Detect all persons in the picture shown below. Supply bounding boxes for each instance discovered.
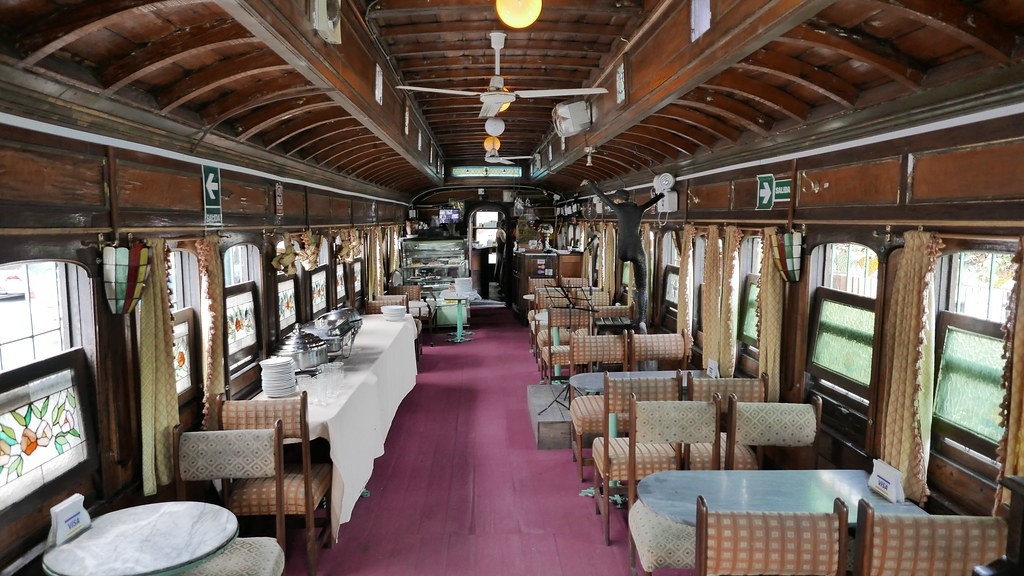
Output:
[581,180,666,334]
[495,219,517,300]
[536,225,543,249]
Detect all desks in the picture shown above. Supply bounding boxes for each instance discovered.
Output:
[40,501,241,576]
[43,289,933,544]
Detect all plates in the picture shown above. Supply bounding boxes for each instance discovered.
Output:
[454,278,473,294]
[259,357,296,397]
[380,305,407,321]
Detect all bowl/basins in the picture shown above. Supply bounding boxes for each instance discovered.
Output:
[405,234,419,238]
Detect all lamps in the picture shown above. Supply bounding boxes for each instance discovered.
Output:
[496,0,542,29]
[587,152,593,166]
[484,137,500,152]
[499,87,511,112]
[653,173,678,212]
[485,117,506,137]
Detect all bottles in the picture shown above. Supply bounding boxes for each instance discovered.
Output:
[576,239,580,247]
[570,238,575,247]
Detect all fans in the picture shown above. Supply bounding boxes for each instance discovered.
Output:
[440,136,534,165]
[395,33,609,117]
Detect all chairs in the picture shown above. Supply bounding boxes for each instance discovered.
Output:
[568,330,629,483]
[387,282,422,356]
[575,290,614,337]
[695,495,849,576]
[542,306,592,384]
[592,369,683,546]
[364,297,405,314]
[531,285,569,362]
[529,275,558,348]
[628,392,721,576]
[592,302,635,372]
[630,330,690,371]
[535,290,578,378]
[669,372,768,470]
[372,292,409,314]
[560,274,590,288]
[851,499,1010,576]
[724,393,822,469]
[173,391,333,576]
[572,286,605,307]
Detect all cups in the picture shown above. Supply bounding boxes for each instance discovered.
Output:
[529,240,537,249]
[443,230,449,236]
[296,362,344,408]
